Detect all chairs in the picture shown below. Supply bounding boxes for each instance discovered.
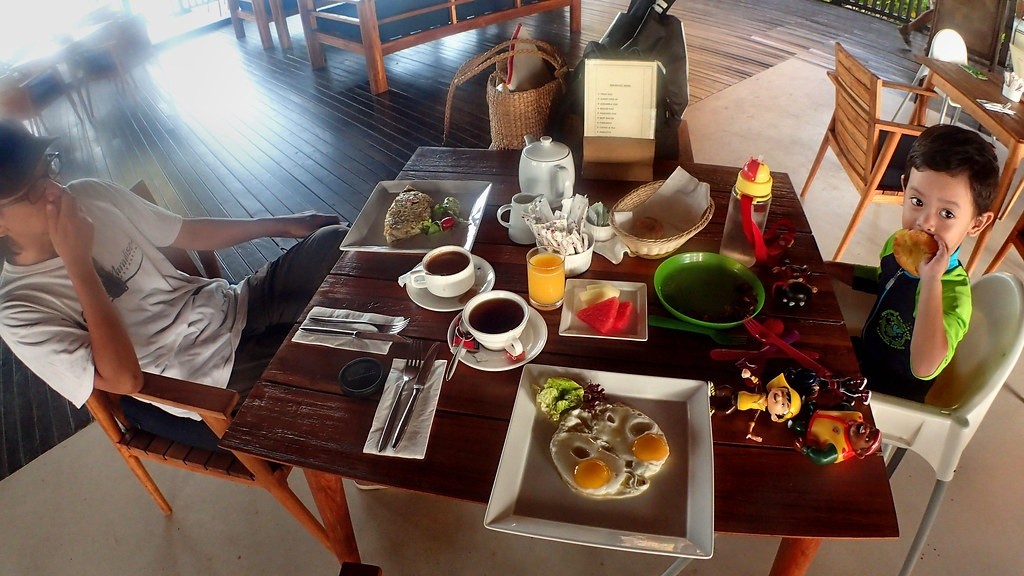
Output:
[78,179,360,566]
[1,38,136,137]
[798,29,1024,576]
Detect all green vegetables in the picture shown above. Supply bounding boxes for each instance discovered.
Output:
[533,377,607,420]
[421,204,458,234]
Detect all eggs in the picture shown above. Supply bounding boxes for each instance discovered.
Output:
[551,402,670,500]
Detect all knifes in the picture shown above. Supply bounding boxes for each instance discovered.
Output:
[391,342,440,451]
[710,349,819,360]
[300,327,414,343]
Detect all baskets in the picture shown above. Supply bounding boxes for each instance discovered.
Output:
[608,180,715,259]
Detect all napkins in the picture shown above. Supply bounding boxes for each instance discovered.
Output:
[977,99,1016,116]
[363,357,447,461]
[293,306,404,356]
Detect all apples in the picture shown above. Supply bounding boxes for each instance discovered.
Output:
[584,285,621,304]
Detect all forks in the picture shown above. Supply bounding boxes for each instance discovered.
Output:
[650,315,747,346]
[309,317,410,334]
[377,340,423,453]
[735,314,824,374]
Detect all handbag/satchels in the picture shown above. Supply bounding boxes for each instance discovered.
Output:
[566,0,689,132]
[443,38,573,150]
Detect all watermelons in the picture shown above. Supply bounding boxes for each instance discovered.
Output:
[578,297,632,334]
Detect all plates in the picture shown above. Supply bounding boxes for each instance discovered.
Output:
[484,364,715,560]
[406,254,495,312]
[447,306,548,371]
[559,278,648,342]
[339,180,492,252]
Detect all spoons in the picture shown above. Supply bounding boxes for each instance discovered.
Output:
[446,324,473,381]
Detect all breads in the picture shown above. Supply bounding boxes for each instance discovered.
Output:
[892,229,938,279]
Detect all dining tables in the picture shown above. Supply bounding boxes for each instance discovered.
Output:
[218,148,902,576]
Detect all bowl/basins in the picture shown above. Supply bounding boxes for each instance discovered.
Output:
[653,252,765,329]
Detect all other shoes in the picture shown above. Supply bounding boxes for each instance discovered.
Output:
[898,24,911,48]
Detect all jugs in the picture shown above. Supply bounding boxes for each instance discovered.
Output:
[498,193,544,245]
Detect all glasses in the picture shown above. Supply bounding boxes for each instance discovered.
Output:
[0,151,63,209]
[91,257,130,298]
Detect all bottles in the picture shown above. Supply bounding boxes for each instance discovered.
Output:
[720,151,773,269]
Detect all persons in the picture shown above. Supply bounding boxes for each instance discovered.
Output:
[0,117,388,489]
[825,123,1000,404]
[708,368,801,442]
[787,396,882,465]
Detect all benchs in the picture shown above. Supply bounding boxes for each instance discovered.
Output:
[221,0,586,94]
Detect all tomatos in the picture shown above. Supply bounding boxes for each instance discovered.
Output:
[439,217,456,230]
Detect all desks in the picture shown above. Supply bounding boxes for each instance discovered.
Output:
[9,20,114,123]
[910,54,1024,283]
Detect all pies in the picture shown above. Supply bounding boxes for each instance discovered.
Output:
[385,185,431,245]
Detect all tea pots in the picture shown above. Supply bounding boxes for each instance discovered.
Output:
[518,133,575,210]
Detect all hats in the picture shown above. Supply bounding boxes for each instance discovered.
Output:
[0,117,60,200]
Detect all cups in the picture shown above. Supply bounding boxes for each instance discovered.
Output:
[526,245,566,310]
[1002,83,1022,103]
[462,290,530,357]
[782,281,812,310]
[535,228,595,277]
[338,358,384,400]
[583,212,615,241]
[410,245,475,298]
